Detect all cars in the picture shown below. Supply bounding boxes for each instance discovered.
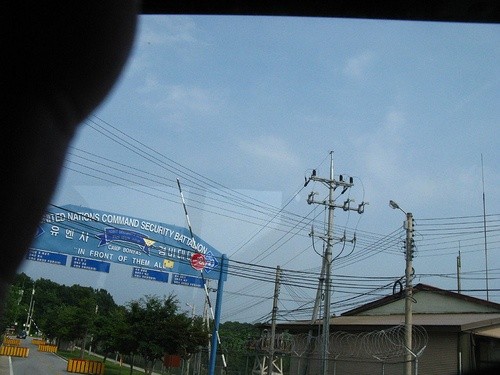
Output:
[17,331,25,338]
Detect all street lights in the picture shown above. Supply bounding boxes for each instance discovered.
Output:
[390,201,415,374]
